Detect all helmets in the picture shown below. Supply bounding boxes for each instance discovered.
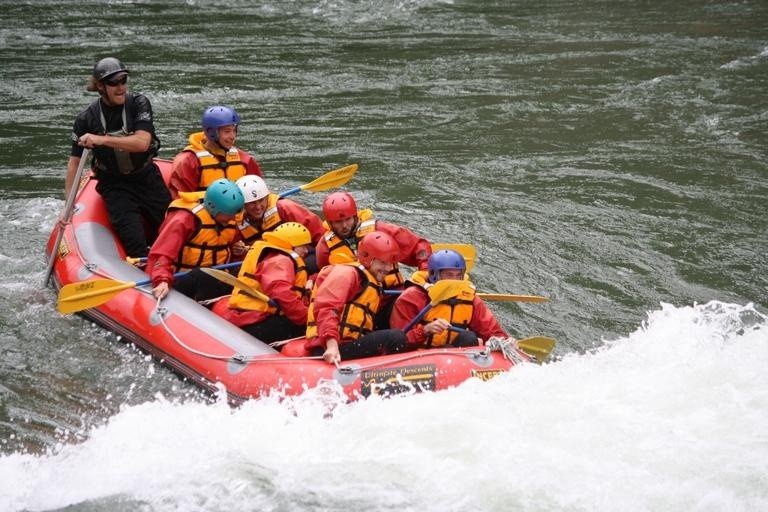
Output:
[204,179,245,217]
[428,250,465,284]
[202,106,240,143]
[272,222,312,247]
[235,175,269,203]
[358,232,401,270]
[323,192,357,230]
[93,57,130,80]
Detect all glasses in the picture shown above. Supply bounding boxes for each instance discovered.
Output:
[106,76,127,86]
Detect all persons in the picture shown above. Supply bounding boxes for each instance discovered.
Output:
[210,221,313,349]
[391,249,508,349]
[315,191,432,272]
[166,105,264,200]
[146,178,246,300]
[58,57,174,268]
[233,174,326,259]
[304,231,408,363]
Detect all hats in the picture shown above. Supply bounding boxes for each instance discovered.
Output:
[108,72,128,82]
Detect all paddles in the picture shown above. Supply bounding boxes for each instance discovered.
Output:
[278,163,356,197]
[448,325,555,364]
[58,262,245,314]
[384,289,548,304]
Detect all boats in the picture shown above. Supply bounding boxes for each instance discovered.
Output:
[44,157,530,409]
